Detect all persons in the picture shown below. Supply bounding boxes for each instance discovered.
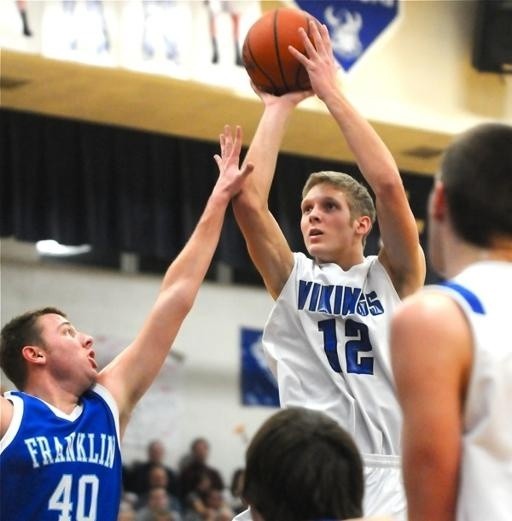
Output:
[244,405,365,521]
[116,440,244,521]
[391,122,512,521]
[2,122,254,521]
[230,20,427,521]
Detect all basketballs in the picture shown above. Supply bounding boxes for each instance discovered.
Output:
[241,9,328,93]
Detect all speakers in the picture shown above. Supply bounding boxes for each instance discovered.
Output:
[472,1,512,72]
